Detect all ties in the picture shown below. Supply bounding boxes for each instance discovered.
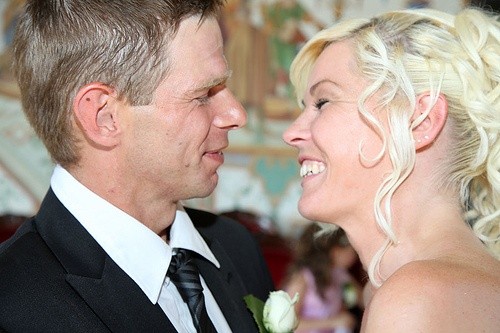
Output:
[168,249,218,333]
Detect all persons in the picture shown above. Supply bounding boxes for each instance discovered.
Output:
[281,5,500,333]
[284,226,365,333]
[0,2,275,333]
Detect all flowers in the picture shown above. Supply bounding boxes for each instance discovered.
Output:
[241,287,301,333]
